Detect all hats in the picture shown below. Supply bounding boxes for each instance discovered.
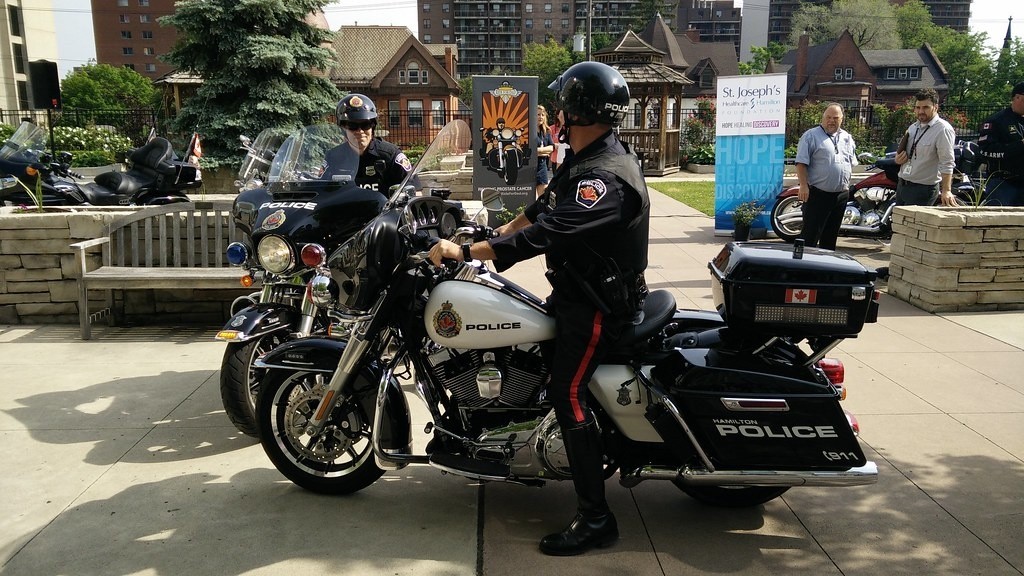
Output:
[1013,81,1024,95]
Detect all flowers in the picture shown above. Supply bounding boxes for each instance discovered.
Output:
[731,199,766,226]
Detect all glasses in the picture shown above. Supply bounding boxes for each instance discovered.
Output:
[342,120,374,131]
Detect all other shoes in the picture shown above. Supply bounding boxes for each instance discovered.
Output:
[875,267,889,279]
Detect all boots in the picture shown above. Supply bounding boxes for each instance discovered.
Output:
[539,418,619,555]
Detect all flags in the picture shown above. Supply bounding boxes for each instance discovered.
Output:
[193,132,201,157]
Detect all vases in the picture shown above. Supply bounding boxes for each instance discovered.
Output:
[733,224,751,241]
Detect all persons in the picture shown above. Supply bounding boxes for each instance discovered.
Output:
[875,89,958,281]
[316,94,423,200]
[430,62,649,556]
[550,109,570,177]
[536,105,555,197]
[794,102,859,251]
[977,81,1024,206]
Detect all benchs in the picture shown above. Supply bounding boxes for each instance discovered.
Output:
[69,201,265,341]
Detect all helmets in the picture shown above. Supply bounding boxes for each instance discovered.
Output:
[555,61,630,125]
[336,94,377,126]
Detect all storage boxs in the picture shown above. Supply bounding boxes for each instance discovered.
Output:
[157,161,204,191]
[707,241,875,336]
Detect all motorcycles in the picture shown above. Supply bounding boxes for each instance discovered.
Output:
[0,120,204,206]
[769,139,986,254]
[215,121,451,435]
[252,119,892,576]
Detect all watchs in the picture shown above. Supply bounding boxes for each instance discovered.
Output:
[461,243,473,263]
